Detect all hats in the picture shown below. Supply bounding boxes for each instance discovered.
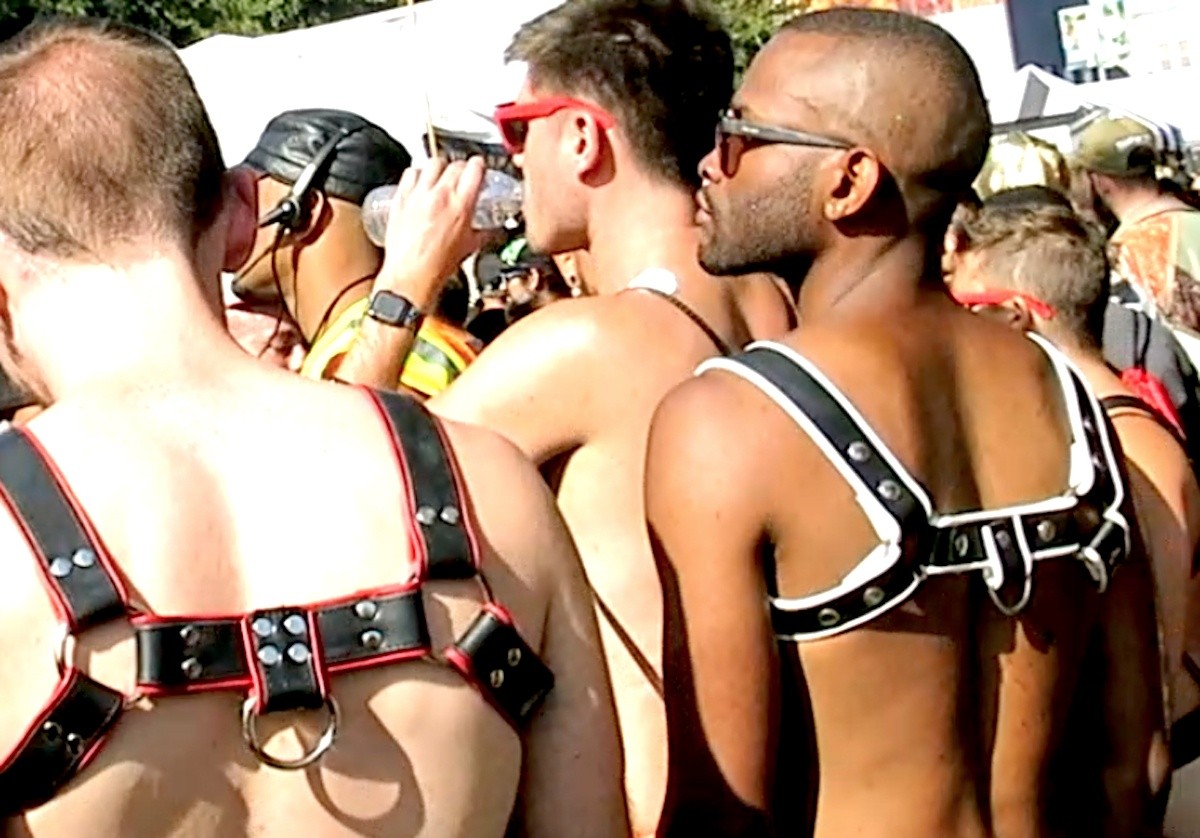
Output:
[1072,118,1157,178]
[245,105,412,206]
[500,238,549,267]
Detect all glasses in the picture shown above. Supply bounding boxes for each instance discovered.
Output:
[499,271,527,283]
[714,112,862,175]
[490,101,617,150]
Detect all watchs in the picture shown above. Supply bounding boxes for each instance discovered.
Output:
[364,289,429,337]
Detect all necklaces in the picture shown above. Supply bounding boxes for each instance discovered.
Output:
[298,272,376,375]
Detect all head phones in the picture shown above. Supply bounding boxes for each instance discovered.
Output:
[277,121,373,228]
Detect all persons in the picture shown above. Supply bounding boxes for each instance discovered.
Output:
[1,1,1200,838]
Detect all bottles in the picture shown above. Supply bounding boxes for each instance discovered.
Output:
[362,170,523,247]
